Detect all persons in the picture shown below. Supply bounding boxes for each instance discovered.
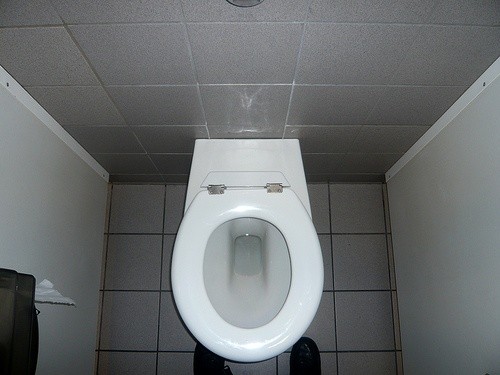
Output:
[193,337,321,375]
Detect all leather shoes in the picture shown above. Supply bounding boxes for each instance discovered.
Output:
[290,337,321,374]
[193,343,224,374]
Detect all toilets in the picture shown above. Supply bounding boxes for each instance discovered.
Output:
[170,139,325,363]
